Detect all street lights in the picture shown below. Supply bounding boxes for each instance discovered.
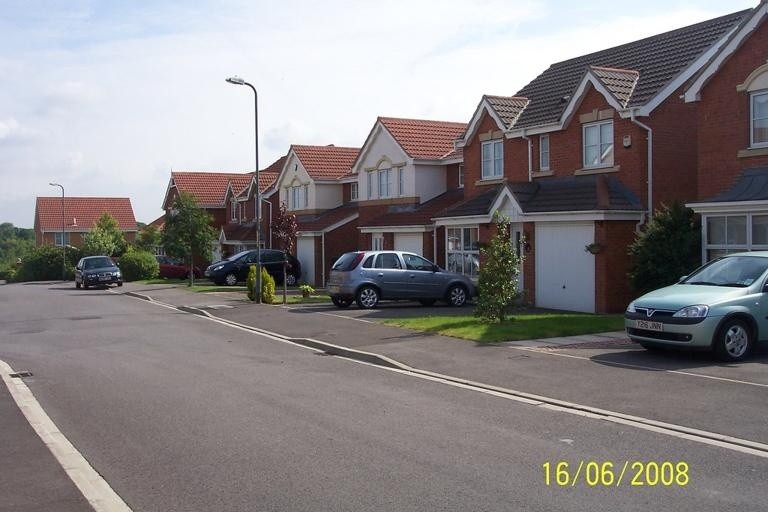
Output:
[49,183,67,281]
[226,76,262,304]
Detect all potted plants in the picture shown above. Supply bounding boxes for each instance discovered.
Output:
[584,242,603,254]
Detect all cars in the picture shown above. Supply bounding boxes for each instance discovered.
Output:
[74,255,123,288]
[204,248,301,286]
[624,251,768,363]
[154,255,202,279]
[327,250,476,308]
